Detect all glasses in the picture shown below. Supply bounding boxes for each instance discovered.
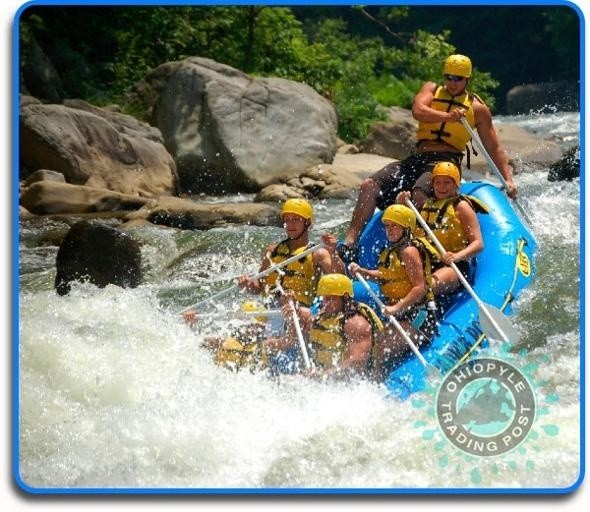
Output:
[445,74,463,82]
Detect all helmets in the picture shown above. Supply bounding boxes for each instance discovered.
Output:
[281,198,312,221]
[381,204,416,234]
[431,162,461,189]
[241,301,268,326]
[316,273,355,299]
[444,54,472,78]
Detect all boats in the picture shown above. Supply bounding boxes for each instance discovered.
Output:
[213,177,539,406]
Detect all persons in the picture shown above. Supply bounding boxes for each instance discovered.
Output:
[396,162,485,300]
[344,52,518,248]
[236,199,344,350]
[181,302,293,372]
[370,205,436,369]
[281,271,380,384]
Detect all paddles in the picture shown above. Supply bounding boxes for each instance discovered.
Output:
[404,196,520,344]
[355,272,443,387]
